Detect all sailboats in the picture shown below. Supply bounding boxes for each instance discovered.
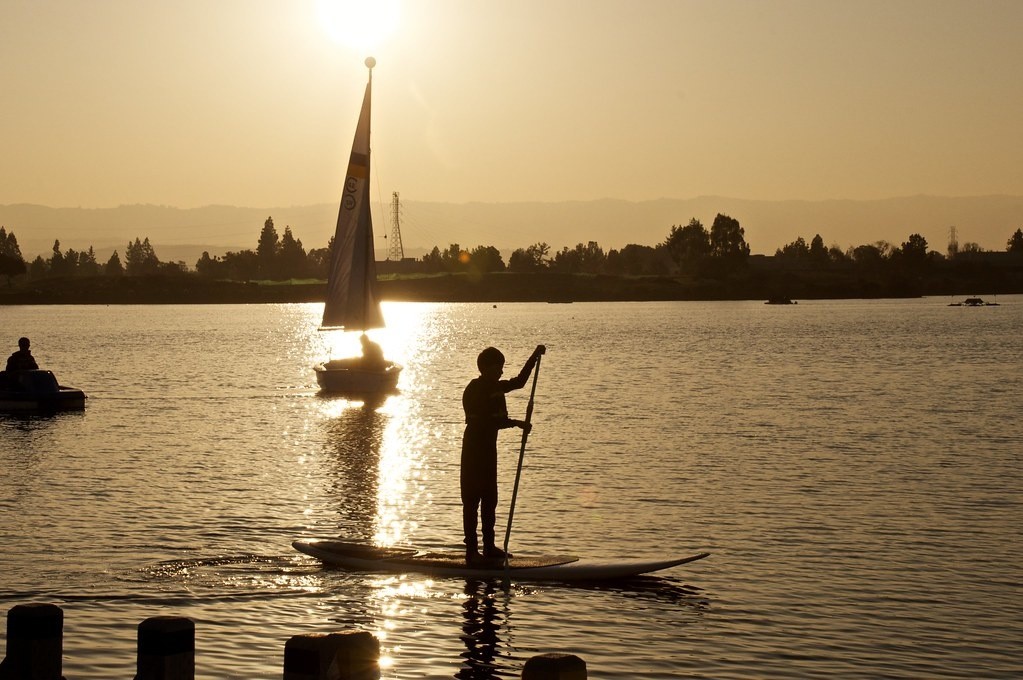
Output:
[310,56,406,390]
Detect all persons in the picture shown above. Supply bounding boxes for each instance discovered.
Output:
[356,334,386,372]
[460,345,546,565]
[5,337,39,371]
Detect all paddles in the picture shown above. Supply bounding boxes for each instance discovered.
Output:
[503,355,543,554]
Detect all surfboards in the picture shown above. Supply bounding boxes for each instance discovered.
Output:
[292,538,712,584]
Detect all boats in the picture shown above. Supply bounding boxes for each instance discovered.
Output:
[0,369,87,416]
